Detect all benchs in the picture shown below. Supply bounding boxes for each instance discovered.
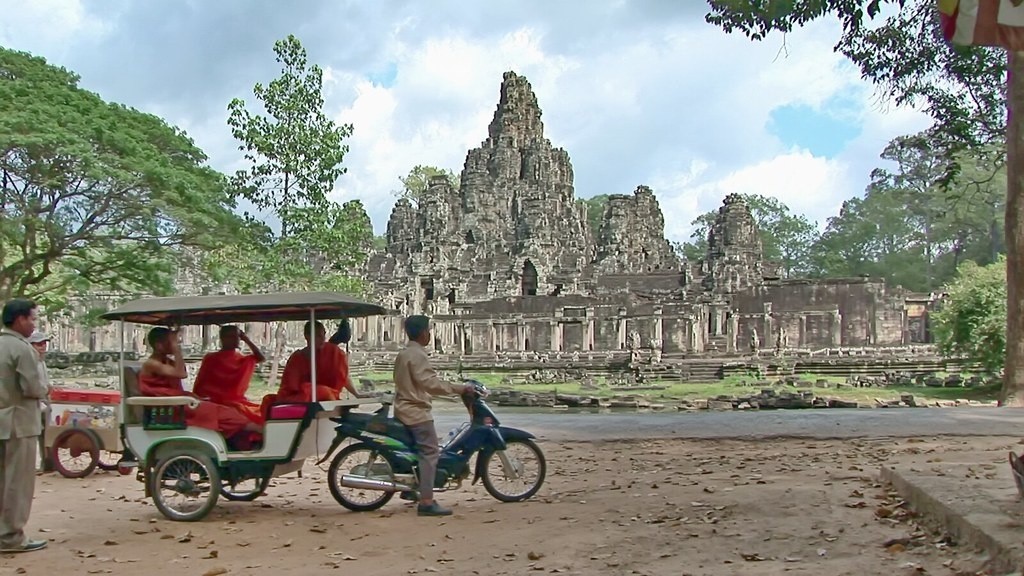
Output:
[123,365,229,453]
[263,393,394,456]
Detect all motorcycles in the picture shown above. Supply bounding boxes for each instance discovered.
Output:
[314,360,548,512]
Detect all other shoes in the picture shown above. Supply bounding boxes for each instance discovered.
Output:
[418,500,452,515]
[400,490,421,500]
[0,538,48,552]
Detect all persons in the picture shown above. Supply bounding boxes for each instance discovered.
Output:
[0,300,50,552]
[394,316,475,516]
[259,321,374,416]
[30,333,57,476]
[192,325,266,451]
[139,327,264,436]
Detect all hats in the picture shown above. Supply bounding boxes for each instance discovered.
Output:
[29,333,51,344]
